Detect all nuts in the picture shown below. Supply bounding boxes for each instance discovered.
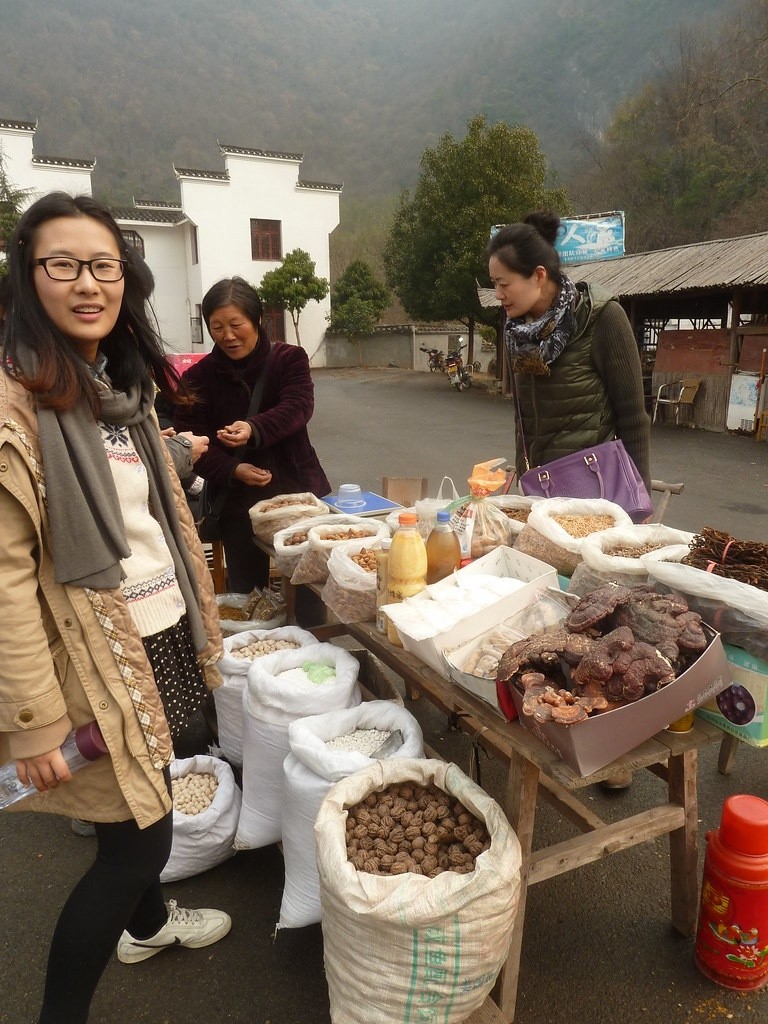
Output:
[251,500,383,626]
[171,773,221,816]
[345,781,490,879]
[227,639,303,661]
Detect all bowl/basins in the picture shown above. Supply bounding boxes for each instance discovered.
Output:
[335,483,366,509]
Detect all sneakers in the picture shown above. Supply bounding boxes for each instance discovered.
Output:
[116,898,232,964]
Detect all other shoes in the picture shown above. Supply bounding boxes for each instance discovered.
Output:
[600,771,633,789]
[71,819,95,836]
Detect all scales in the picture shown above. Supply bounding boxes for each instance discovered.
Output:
[319,491,406,524]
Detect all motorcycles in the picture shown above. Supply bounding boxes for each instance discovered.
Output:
[419,342,447,373]
[442,336,474,393]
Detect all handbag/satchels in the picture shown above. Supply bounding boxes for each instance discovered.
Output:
[413,475,461,548]
[195,478,224,542]
[518,436,654,524]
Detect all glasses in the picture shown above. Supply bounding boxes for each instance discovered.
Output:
[28,254,129,283]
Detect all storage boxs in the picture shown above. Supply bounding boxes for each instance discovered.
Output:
[392,545,768,776]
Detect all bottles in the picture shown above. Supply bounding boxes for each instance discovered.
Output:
[692,795,768,990]
[0,719,110,810]
[375,538,393,634]
[387,513,428,645]
[426,511,461,584]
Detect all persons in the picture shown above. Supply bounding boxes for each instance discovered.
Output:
[1,192,232,1023]
[486,212,650,787]
[171,276,331,594]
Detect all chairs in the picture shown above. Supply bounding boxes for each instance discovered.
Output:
[653,380,701,426]
